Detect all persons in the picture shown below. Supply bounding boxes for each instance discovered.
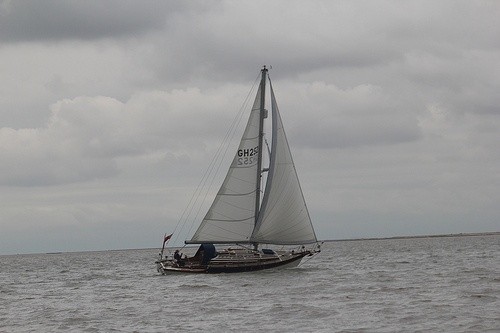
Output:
[174,250,183,261]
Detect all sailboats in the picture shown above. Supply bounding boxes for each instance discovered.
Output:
[155,65,322,276]
